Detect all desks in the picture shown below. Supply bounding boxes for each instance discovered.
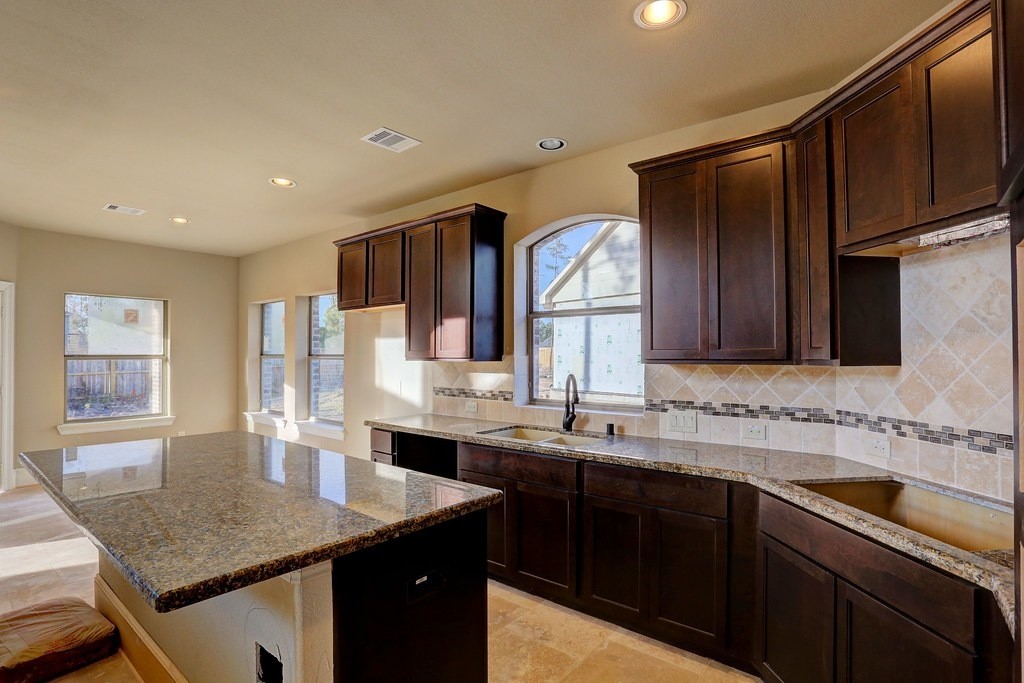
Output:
[14,430,505,683]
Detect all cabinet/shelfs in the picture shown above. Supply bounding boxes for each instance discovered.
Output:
[370,428,458,480]
[333,200,506,364]
[457,440,581,614]
[628,0,1024,365]
[751,482,994,683]
[582,459,730,666]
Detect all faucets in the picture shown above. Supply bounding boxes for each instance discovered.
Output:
[562,374,580,432]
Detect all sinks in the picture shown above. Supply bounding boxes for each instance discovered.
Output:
[541,431,609,447]
[476,424,562,442]
